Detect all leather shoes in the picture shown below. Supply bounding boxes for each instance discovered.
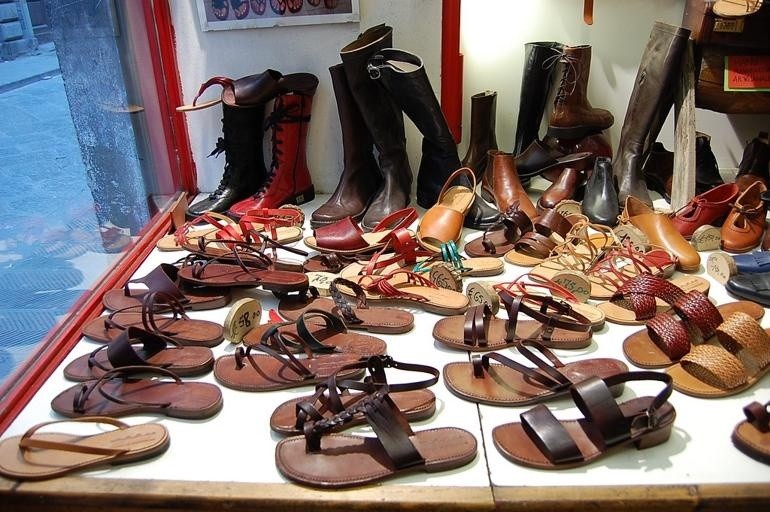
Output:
[731,251,770,273]
[724,273,770,310]
[721,180,769,254]
[616,195,701,272]
[582,156,621,228]
[671,183,740,239]
[536,167,589,212]
[638,131,770,204]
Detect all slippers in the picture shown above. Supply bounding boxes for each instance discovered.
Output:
[222,69,320,110]
[65,326,216,383]
[176,77,235,113]
[443,339,631,408]
[513,138,594,179]
[51,365,225,421]
[334,227,506,318]
[104,230,311,314]
[82,291,226,349]
[465,200,680,334]
[211,0,337,20]
[595,271,770,465]
[1,415,171,482]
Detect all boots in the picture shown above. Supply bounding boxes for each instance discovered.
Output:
[340,24,414,234]
[548,45,615,139]
[311,64,383,232]
[512,42,564,192]
[186,102,269,227]
[461,90,499,187]
[612,22,692,213]
[228,94,316,225]
[368,48,508,232]
[481,149,539,222]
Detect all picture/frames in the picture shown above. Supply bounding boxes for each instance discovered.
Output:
[195,0,360,32]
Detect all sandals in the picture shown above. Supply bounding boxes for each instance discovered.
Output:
[493,371,677,471]
[433,289,594,351]
